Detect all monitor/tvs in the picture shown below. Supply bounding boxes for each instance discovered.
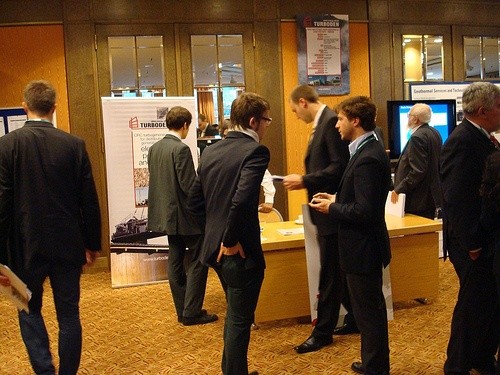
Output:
[387,99,456,159]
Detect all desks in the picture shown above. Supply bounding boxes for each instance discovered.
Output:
[251,212,442,330]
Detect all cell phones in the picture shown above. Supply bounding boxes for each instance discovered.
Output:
[270,175,287,182]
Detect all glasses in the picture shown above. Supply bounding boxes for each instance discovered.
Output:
[407,112,414,117]
[258,116,273,124]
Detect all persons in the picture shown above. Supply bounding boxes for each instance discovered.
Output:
[259,169,277,213]
[0,80,101,375]
[282,84,361,353]
[146,106,219,326]
[197,92,270,375]
[309,95,390,375]
[440,81,500,375]
[197,113,231,156]
[391,103,443,220]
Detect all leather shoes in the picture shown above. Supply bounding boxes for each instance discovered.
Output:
[292,334,333,352]
[333,323,363,336]
[177,310,219,326]
[352,361,389,375]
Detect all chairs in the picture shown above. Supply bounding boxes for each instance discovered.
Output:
[258,206,284,222]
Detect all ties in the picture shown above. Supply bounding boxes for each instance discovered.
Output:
[489,135,500,147]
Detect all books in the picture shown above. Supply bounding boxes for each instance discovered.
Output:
[385,191,407,219]
[267,175,285,180]
[0,263,33,313]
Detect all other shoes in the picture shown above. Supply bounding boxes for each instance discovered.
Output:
[440,359,500,374]
[240,371,260,375]
